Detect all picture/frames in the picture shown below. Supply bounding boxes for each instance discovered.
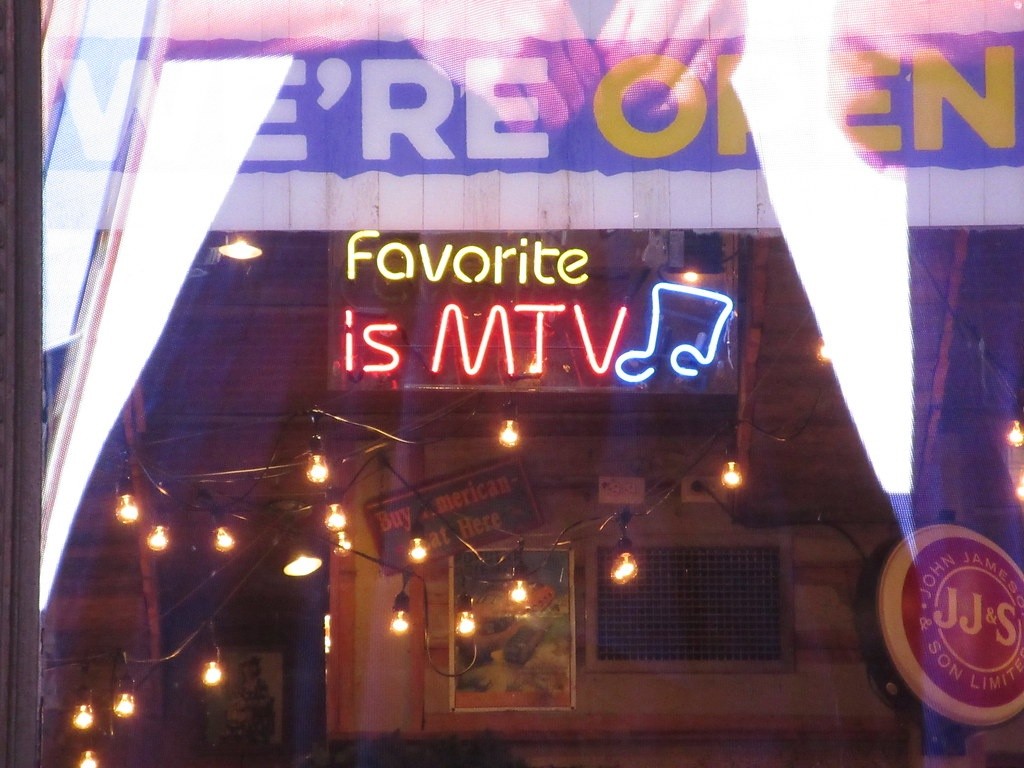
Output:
[363,453,545,576]
[449,546,577,712]
[191,634,295,760]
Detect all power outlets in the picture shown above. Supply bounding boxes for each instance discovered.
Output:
[599,475,645,504]
[680,476,728,504]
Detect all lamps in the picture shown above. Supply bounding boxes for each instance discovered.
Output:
[280,530,322,578]
[218,232,263,260]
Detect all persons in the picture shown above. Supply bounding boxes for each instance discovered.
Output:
[44,2,1024,768]
